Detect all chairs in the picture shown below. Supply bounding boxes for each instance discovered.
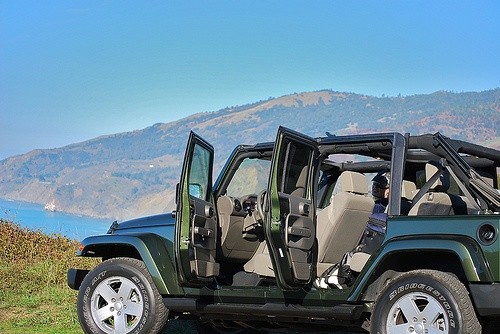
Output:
[385,181,418,219]
[409,162,452,218]
[258,170,375,266]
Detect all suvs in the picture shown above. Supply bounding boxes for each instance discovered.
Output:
[67,125,500,334]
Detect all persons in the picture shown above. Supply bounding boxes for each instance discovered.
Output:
[372,171,390,207]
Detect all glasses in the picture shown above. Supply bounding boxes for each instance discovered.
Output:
[372,181,388,191]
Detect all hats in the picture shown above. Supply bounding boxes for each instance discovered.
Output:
[371,171,392,187]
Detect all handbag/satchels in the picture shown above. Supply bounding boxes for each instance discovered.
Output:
[312,254,357,291]
